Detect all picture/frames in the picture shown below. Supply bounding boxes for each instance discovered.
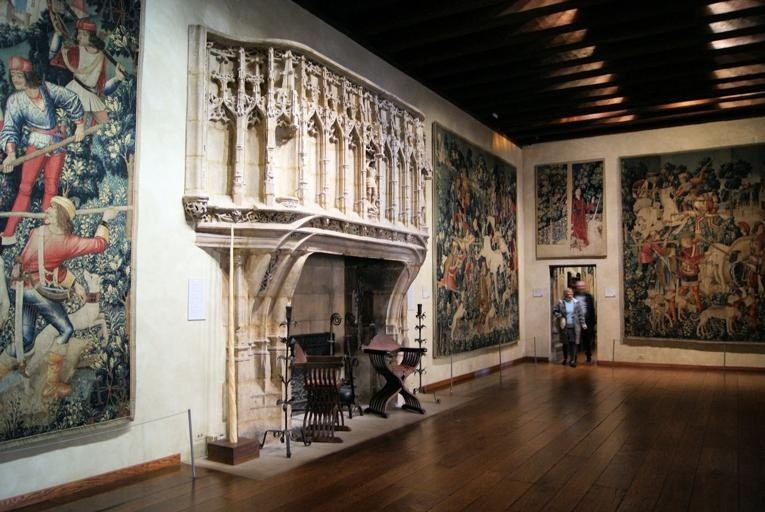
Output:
[432,121,520,360]
[620,143,764,354]
[535,158,607,259]
[0,2,146,464]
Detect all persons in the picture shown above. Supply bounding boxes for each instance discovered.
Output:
[558,280,596,363]
[551,287,587,367]
[437,145,518,332]
[631,166,765,330]
[571,184,597,251]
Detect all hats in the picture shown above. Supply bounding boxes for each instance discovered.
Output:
[75,19,97,32]
[7,57,33,72]
[51,196,74,218]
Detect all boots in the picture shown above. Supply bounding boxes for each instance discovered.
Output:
[42,350,72,396]
[562,351,578,367]
[0,349,34,378]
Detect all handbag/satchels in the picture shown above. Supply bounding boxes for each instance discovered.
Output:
[560,317,567,330]
